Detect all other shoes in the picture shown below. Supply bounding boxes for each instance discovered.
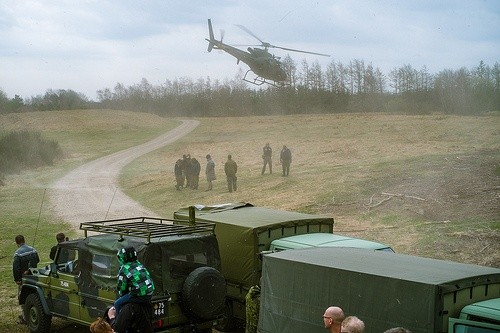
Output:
[19,315,26,324]
[176,186,180,191]
[193,186,198,190]
[205,189,210,191]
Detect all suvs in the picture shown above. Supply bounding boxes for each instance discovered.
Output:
[19,217,227,333]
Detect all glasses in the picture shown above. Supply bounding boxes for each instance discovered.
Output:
[321,315,331,321]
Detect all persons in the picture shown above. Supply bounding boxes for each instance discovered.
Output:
[12,235,39,324]
[174,153,201,191]
[225,154,237,193]
[280,145,291,177]
[206,155,216,191]
[322,305,344,333]
[114,246,155,317]
[262,143,272,174]
[90,301,156,333]
[50,233,69,265]
[341,315,365,333]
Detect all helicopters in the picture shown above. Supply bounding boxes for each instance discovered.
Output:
[204,18,331,88]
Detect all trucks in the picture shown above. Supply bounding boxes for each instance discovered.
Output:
[171,204,397,330]
[255,248,500,333]
